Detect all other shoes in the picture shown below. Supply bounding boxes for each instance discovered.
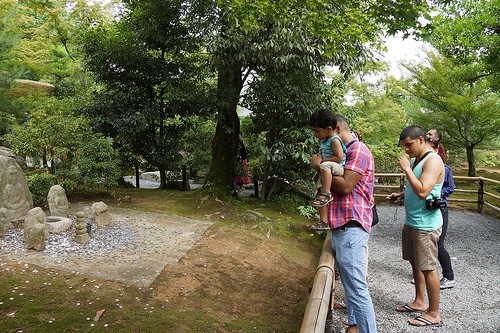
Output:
[346,324,359,333]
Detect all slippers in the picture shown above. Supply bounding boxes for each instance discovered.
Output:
[396,305,426,312]
[408,316,443,327]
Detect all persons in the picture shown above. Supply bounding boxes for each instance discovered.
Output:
[310,114,378,333]
[426,128,442,142]
[309,109,347,230]
[236,130,252,197]
[386,125,445,327]
[411,138,455,289]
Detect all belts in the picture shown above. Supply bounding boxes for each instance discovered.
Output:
[336,220,363,230]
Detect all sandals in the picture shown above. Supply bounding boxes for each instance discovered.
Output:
[311,221,330,231]
[313,192,334,206]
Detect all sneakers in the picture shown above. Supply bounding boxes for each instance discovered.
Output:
[411,279,415,284]
[440,277,454,289]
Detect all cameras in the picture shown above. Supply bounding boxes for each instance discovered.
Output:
[426,197,446,210]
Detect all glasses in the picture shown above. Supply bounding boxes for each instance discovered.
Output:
[426,138,438,148]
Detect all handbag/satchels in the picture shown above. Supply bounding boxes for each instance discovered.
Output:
[371,201,379,227]
